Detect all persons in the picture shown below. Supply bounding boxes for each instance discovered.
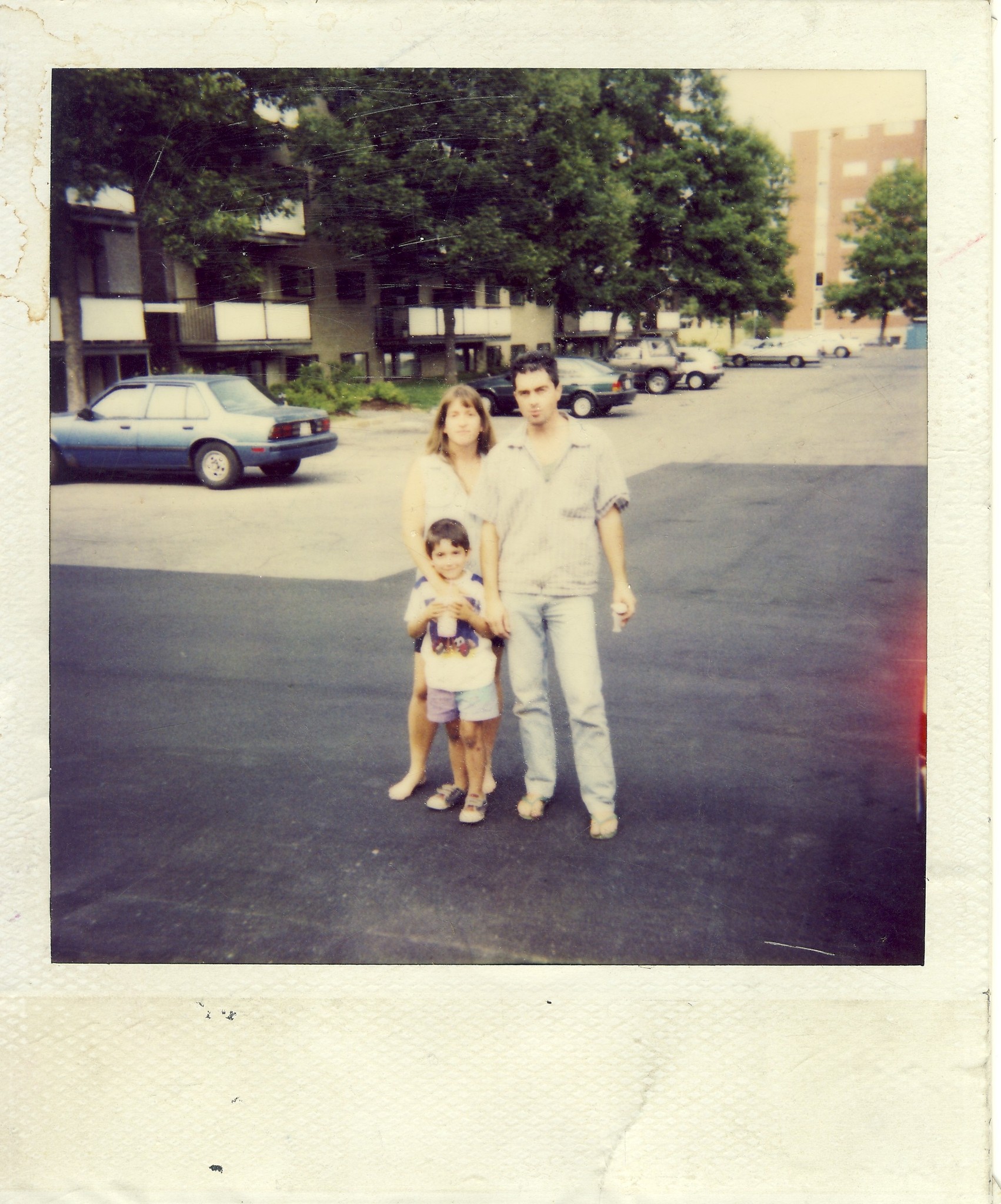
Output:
[387,384,504,802]
[407,519,500,822]
[468,352,637,838]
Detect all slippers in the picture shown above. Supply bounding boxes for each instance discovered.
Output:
[588,814,619,840]
[517,796,549,820]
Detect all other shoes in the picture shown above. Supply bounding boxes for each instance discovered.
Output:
[458,793,489,824]
[426,783,465,809]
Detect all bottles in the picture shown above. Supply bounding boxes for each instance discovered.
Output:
[437,610,457,638]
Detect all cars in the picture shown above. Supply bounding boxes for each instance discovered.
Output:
[461,356,639,420]
[50,374,338,490]
[723,335,826,368]
[678,345,727,390]
[806,332,866,358]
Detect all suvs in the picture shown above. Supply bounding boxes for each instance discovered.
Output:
[591,336,687,395]
